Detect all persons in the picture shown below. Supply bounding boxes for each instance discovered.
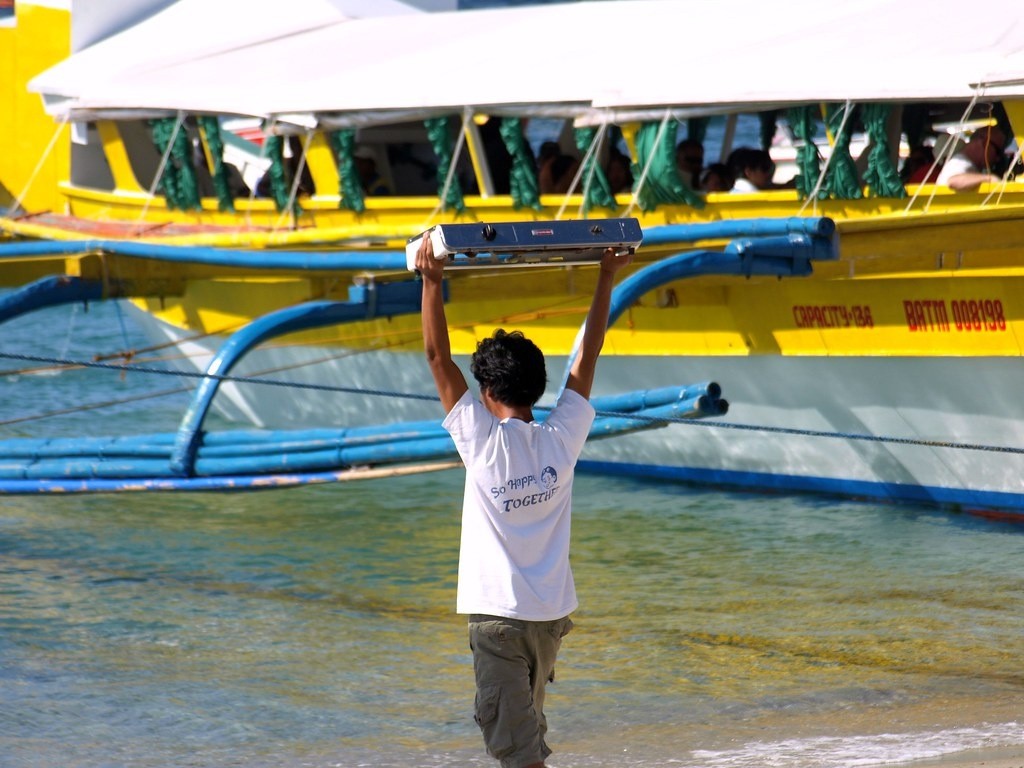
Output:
[417,229,641,767]
[193,123,1005,197]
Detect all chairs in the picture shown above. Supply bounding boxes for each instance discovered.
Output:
[540,155,579,194]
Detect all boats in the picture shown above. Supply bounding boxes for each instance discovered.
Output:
[0,0,1022,520]
